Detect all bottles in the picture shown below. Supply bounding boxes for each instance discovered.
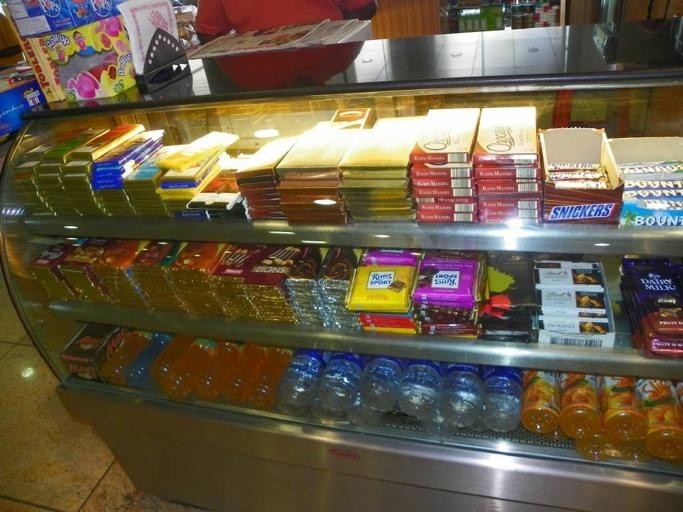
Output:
[102,328,682,457]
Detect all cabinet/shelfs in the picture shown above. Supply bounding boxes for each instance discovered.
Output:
[21,138,683,476]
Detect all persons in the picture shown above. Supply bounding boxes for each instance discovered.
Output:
[208,45,364,89]
[192,1,376,48]
